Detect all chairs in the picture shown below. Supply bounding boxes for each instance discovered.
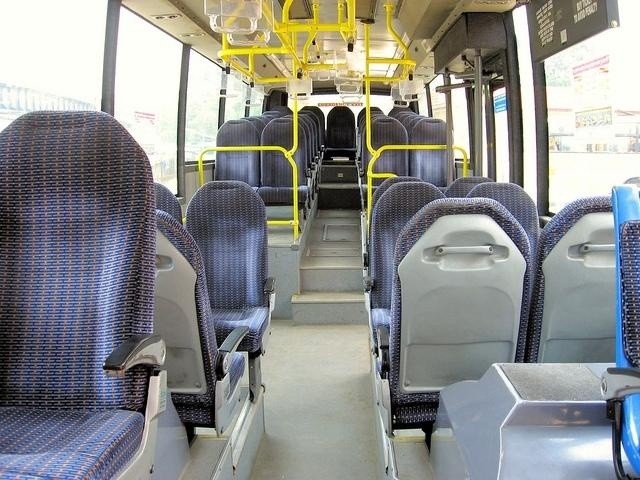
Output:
[1,105,640,479]
